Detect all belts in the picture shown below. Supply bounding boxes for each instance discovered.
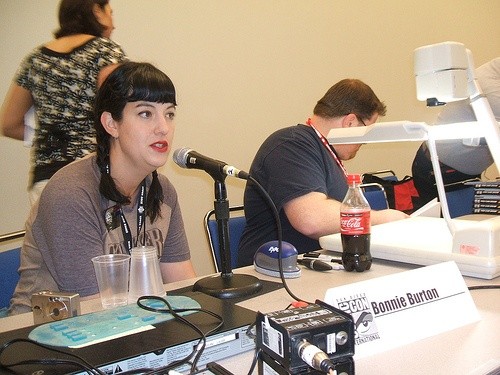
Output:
[421,142,457,173]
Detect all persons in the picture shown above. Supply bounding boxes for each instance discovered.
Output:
[412,56,500,211]
[0,0,130,207]
[238,79,410,268]
[7,61,196,316]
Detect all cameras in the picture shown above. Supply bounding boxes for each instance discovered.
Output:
[32,291,81,325]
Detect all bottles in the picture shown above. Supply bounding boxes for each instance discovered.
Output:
[340,174,374,271]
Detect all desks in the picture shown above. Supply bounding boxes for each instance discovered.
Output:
[0,213,500,375]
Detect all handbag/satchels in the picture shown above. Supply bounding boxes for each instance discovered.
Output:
[362,173,420,213]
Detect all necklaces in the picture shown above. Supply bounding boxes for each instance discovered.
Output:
[106,161,145,255]
[306,119,348,180]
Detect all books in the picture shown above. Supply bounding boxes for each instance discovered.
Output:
[464,178,500,214]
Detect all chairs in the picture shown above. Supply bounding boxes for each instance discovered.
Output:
[204,205,249,272]
[358,170,399,210]
[0,230,27,319]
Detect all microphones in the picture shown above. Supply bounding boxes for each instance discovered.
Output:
[173,147,251,182]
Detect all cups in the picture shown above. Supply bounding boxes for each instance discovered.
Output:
[91,254,130,309]
[130,246,168,303]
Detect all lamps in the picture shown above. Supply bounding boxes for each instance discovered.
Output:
[324,121,500,281]
[414,40,500,170]
